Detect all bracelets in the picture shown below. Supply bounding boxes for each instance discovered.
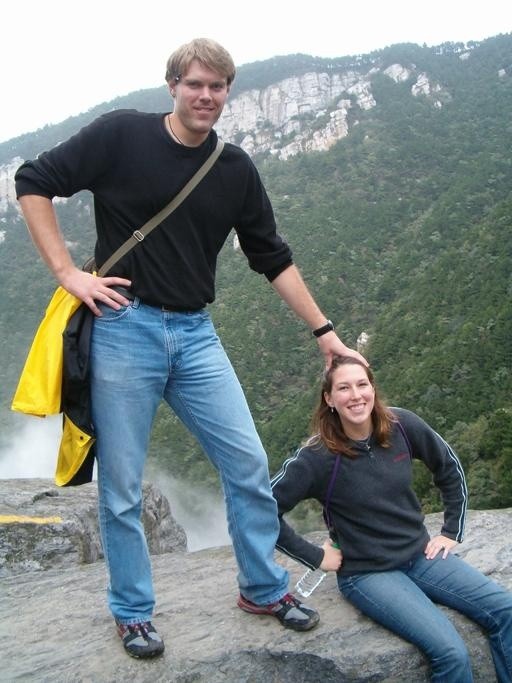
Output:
[310,318,335,338]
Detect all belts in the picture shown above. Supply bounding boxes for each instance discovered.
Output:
[113,285,174,311]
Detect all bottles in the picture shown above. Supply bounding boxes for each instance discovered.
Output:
[295,544,339,599]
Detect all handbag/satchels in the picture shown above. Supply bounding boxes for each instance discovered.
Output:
[82,255,99,274]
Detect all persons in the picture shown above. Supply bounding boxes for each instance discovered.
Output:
[15,37,368,661]
[264,351,510,682]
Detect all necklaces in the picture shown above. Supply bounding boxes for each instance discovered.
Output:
[358,433,371,448]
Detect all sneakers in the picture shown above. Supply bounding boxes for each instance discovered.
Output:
[237,590,319,631]
[114,618,164,660]
